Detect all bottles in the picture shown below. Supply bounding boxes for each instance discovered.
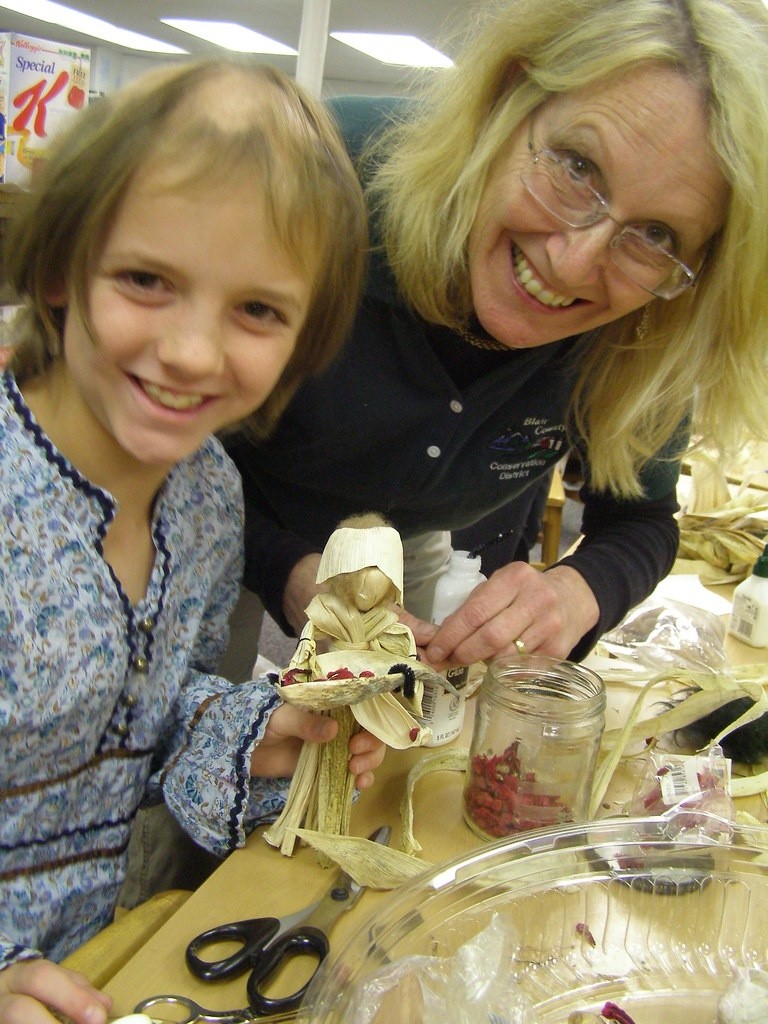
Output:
[412,550,489,747]
[728,544,768,647]
[461,655,606,846]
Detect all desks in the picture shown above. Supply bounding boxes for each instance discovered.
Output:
[31,466,768,1024]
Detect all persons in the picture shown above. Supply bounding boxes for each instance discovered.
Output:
[264,520,427,868]
[0,0,768,1024]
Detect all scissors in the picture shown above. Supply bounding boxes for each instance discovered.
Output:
[184,824,392,1014]
[132,989,342,1024]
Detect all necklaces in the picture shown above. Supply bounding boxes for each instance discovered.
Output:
[459,330,515,352]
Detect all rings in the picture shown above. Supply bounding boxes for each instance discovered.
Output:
[515,640,531,660]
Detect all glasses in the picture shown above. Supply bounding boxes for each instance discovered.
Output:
[521,112,722,301]
